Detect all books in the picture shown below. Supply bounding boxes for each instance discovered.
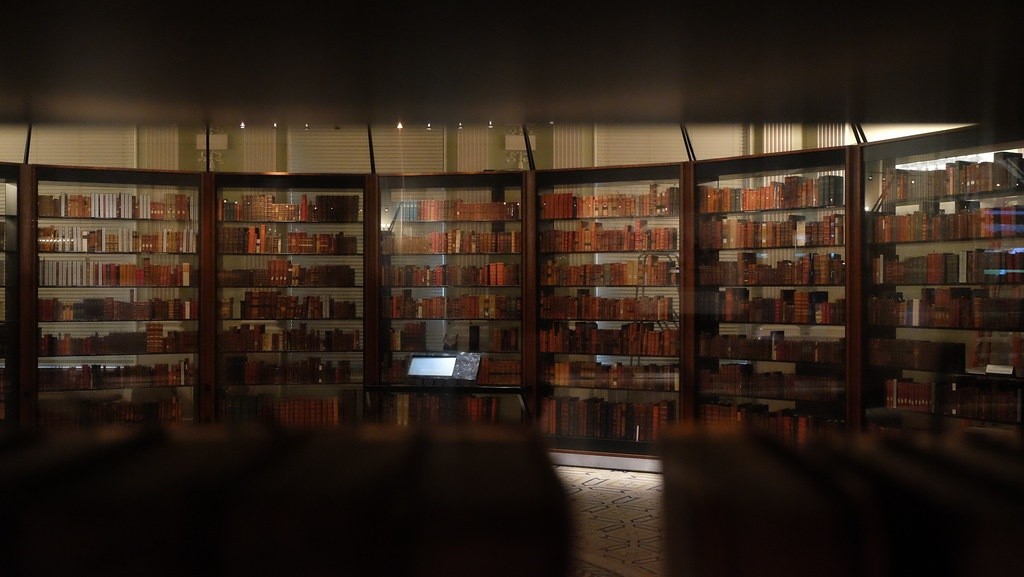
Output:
[535,152,1024,442]
[35,193,522,428]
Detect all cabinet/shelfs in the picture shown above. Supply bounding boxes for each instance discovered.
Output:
[0,123,1024,455]
